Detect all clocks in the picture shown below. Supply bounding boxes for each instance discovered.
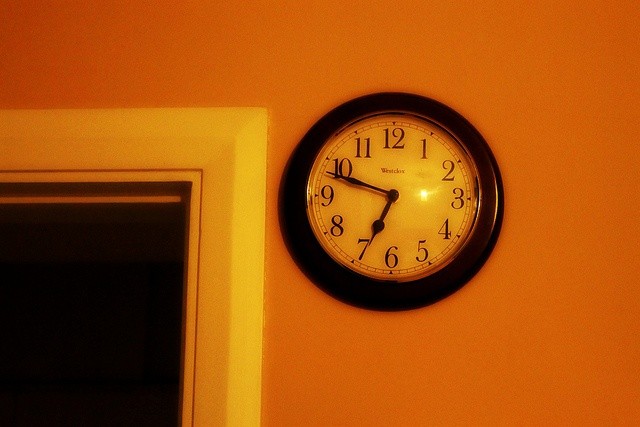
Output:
[278,92,504,311]
